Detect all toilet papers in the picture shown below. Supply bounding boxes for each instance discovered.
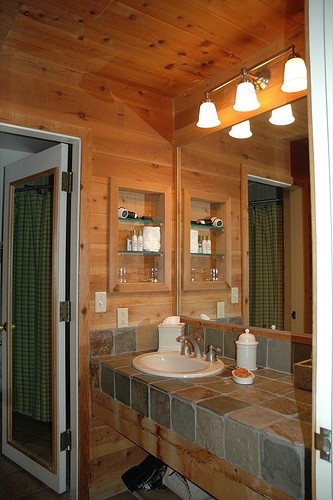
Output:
[190,229,199,252]
[142,225,160,252]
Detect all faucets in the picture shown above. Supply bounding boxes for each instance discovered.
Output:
[176,335,203,359]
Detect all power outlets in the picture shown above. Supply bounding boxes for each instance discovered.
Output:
[217,302,224,318]
[116,307,129,327]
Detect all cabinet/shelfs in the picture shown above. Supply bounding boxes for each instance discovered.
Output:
[182,187,233,290]
[107,176,171,292]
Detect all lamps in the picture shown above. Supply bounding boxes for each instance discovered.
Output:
[268,104,295,126]
[228,121,252,140]
[195,45,307,130]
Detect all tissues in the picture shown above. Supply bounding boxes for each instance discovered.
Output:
[156,315,187,354]
[199,313,216,322]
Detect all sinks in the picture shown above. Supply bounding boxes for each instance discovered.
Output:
[131,351,224,379]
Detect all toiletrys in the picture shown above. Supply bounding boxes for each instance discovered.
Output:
[130,229,138,251]
[207,235,212,255]
[138,230,145,252]
[126,234,132,251]
[234,366,253,379]
[198,236,203,253]
[202,236,207,253]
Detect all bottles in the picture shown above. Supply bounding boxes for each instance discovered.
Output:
[151,268,154,282]
[212,267,216,281]
[154,265,158,282]
[191,265,194,282]
[123,267,127,283]
[216,268,219,280]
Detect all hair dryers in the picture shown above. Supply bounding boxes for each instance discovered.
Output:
[196,216,223,228]
[117,207,138,219]
